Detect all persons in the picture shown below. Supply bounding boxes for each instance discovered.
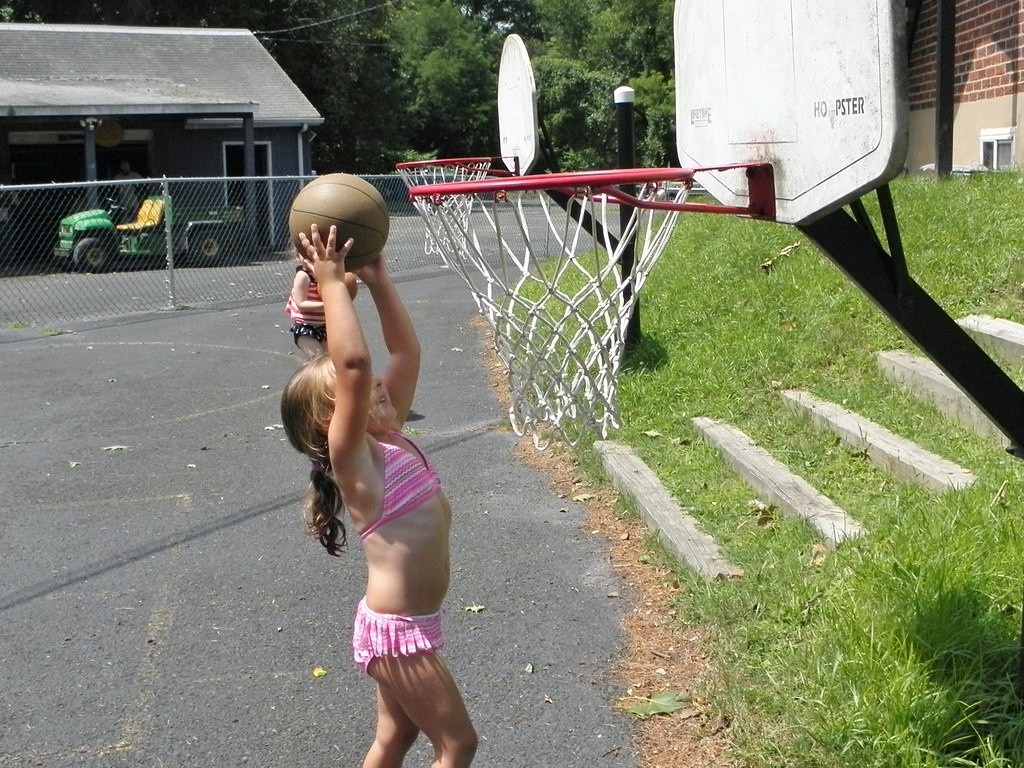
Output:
[282,224,478,767]
[285,240,328,362]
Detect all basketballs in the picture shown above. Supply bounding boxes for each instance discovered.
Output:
[287,172,390,273]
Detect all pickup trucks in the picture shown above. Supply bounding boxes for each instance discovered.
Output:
[54,193,247,274]
